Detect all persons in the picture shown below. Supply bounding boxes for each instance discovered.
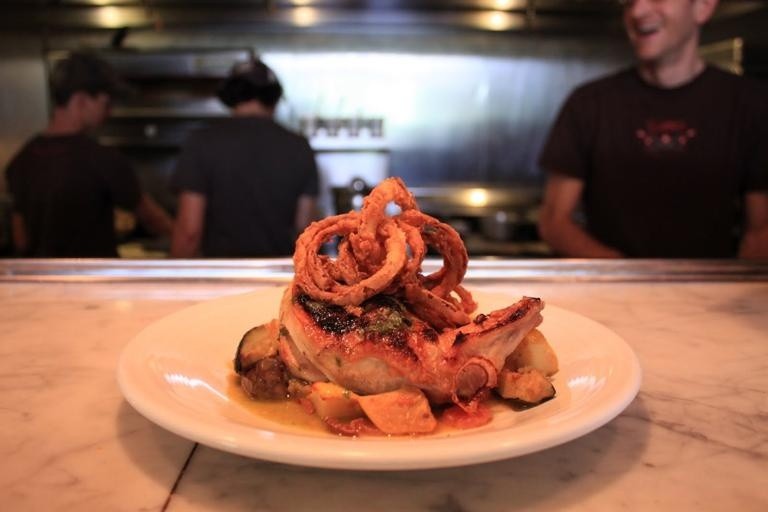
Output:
[535,1,766,262]
[172,59,318,259]
[4,50,173,262]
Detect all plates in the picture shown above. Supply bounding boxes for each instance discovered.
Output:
[117,284,641,472]
